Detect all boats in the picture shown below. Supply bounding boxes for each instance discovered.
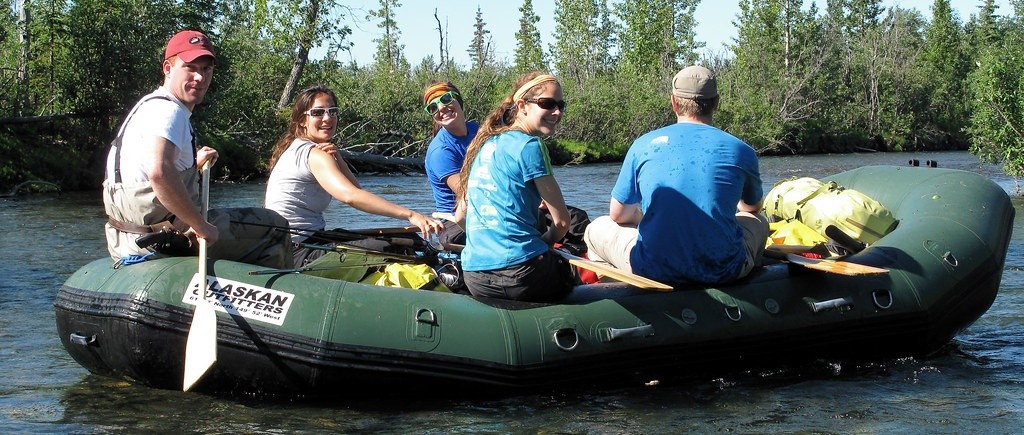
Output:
[51,160,1014,408]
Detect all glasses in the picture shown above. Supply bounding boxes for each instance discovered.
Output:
[525,97,567,113]
[424,90,459,115]
[303,107,340,117]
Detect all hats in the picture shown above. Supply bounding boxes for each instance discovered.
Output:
[671,65,718,100]
[423,81,464,111]
[165,30,216,63]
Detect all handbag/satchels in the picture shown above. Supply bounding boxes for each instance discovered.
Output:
[761,176,899,253]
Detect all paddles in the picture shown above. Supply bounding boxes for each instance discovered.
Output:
[555,247,675,293]
[183,156,218,394]
[331,223,466,253]
[764,249,891,277]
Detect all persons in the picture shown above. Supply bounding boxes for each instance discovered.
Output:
[583,66,769,285]
[421,80,480,245]
[102,30,295,271]
[264,85,445,268]
[455,73,574,301]
